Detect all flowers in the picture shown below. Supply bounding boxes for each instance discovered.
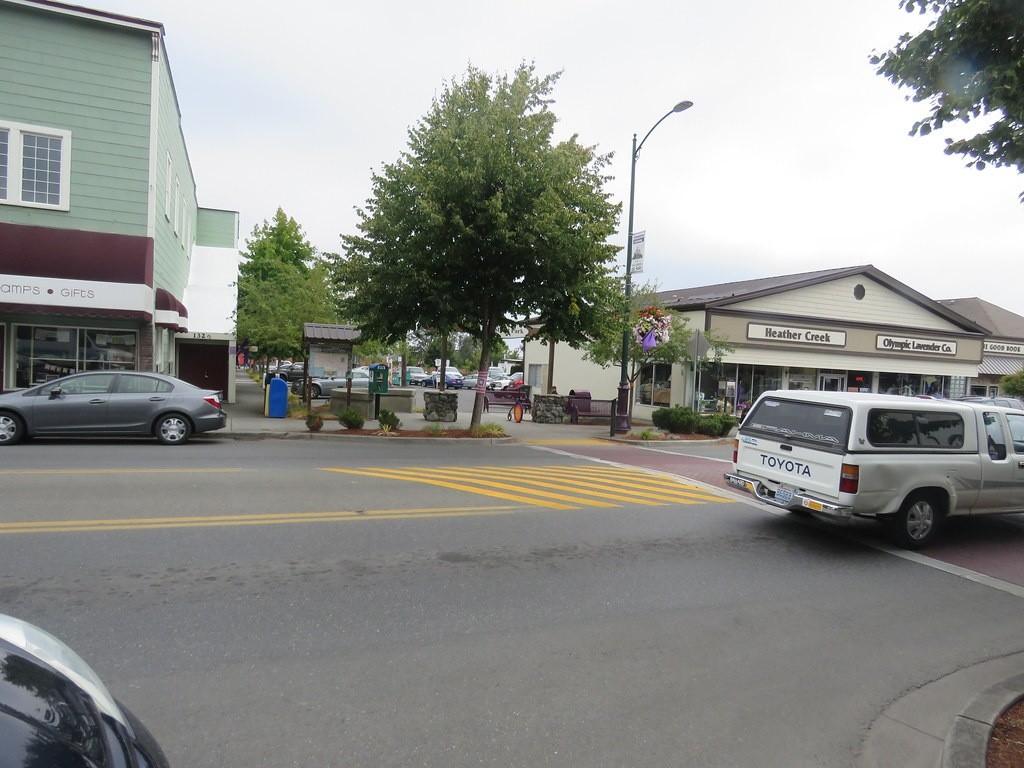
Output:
[632,306,672,347]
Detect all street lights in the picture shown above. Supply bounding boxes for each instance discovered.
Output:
[614,101,693,435]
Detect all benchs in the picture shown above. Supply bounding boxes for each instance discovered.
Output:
[484,391,532,413]
[568,397,617,424]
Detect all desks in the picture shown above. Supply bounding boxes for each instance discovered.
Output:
[696,400,714,411]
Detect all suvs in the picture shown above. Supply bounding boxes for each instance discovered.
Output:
[949,395,1024,411]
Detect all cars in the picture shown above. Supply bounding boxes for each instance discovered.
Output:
[420,373,464,390]
[488,371,523,399]
[407,367,429,385]
[0,369,228,447]
[265,360,304,384]
[462,374,494,390]
[0,612,170,768]
[291,371,394,400]
[432,366,463,377]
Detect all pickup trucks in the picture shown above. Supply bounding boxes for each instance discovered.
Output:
[723,389,1024,551]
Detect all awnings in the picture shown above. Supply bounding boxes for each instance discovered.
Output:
[154,289,189,333]
[0,222,154,327]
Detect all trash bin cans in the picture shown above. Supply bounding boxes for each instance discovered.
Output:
[519,385,530,401]
[392,371,411,386]
[265,378,289,418]
